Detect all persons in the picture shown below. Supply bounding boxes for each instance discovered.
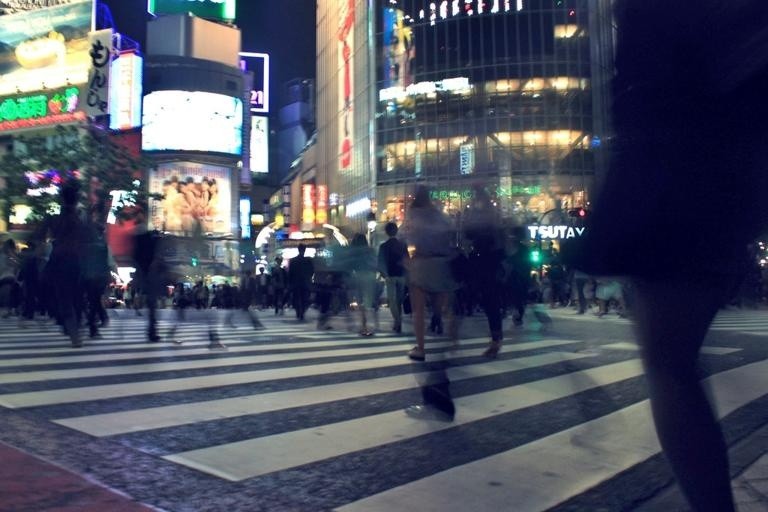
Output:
[41,187,90,347]
[558,3,768,508]
[393,182,457,362]
[0,219,627,351]
[463,182,507,358]
